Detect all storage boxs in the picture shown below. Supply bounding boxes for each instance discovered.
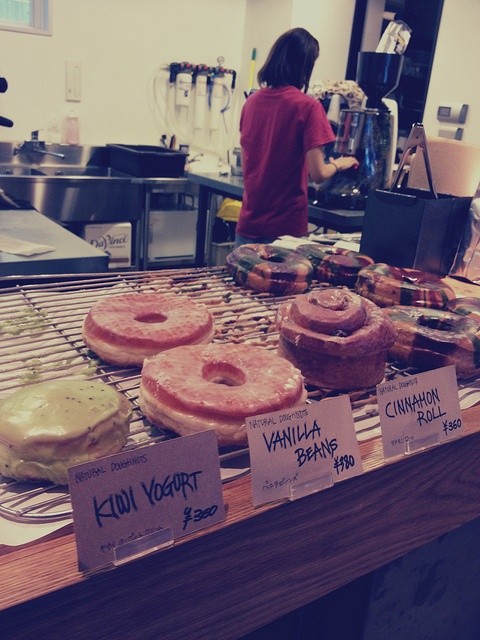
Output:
[360,185,470,280]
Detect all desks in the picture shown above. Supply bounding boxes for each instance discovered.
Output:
[187,167,368,268]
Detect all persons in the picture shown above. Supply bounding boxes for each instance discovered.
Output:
[233,26,360,252]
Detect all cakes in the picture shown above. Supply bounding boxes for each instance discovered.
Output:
[1,375,133,490]
[274,284,397,388]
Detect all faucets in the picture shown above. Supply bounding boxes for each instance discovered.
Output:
[13,127,66,159]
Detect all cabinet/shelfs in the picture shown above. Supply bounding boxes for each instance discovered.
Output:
[1,266,479,640]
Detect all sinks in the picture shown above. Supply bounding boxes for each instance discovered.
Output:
[39,164,142,177]
[0,165,48,176]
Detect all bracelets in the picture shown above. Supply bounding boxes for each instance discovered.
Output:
[330,162,339,173]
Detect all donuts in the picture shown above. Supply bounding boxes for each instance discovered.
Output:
[451,297,480,319]
[299,244,374,285]
[85,295,216,366]
[384,303,479,381]
[136,340,305,450]
[356,264,457,312]
[225,242,314,295]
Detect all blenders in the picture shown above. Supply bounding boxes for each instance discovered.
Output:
[313,52,404,210]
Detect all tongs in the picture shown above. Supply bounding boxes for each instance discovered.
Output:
[390,123,439,199]
